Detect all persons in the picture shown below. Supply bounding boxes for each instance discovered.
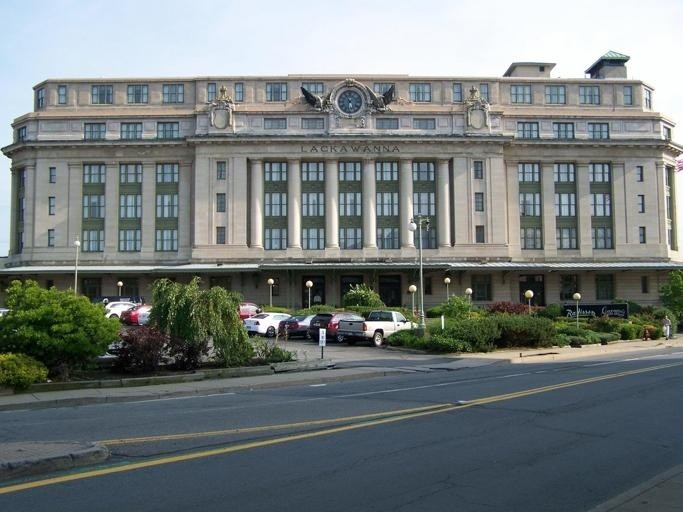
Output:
[128,296,134,302]
[662,316,671,340]
[133,295,140,303]
[103,300,108,306]
[141,296,145,305]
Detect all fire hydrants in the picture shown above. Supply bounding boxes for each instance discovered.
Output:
[644,329,649,341]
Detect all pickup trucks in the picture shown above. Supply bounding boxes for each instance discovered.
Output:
[335,310,419,347]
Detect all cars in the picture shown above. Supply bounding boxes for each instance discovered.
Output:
[103,302,364,343]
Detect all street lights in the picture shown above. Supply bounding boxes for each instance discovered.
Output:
[306,280,313,309]
[465,288,473,320]
[525,289,534,315]
[73,240,80,295]
[409,285,417,317]
[117,280,123,296]
[267,278,274,307]
[573,293,581,329]
[408,215,431,330]
[444,277,450,305]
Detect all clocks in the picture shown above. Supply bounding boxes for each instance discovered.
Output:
[334,86,365,116]
